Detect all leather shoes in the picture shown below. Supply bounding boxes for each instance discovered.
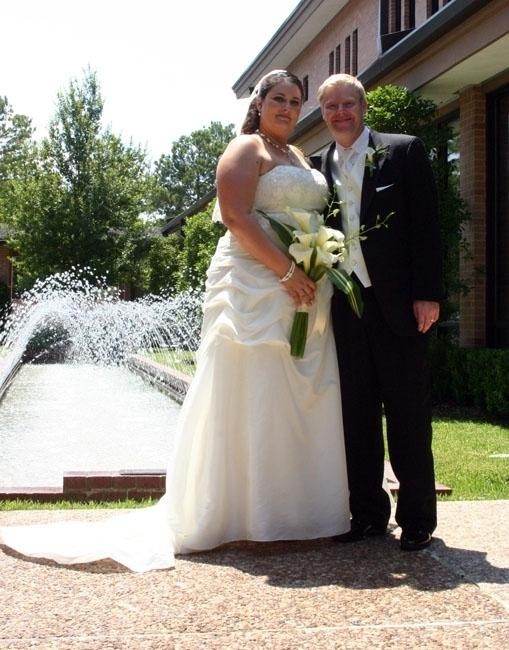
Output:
[400,528,432,551]
[331,519,387,543]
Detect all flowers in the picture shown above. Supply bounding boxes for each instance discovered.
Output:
[363,143,392,176]
[253,183,396,361]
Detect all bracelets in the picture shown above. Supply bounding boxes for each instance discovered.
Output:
[279,260,296,283]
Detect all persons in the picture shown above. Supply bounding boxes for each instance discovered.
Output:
[309,74,444,551]
[0,70,350,572]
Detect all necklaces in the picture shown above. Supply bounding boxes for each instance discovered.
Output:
[255,129,295,165]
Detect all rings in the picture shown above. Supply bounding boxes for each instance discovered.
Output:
[430,319,435,323]
